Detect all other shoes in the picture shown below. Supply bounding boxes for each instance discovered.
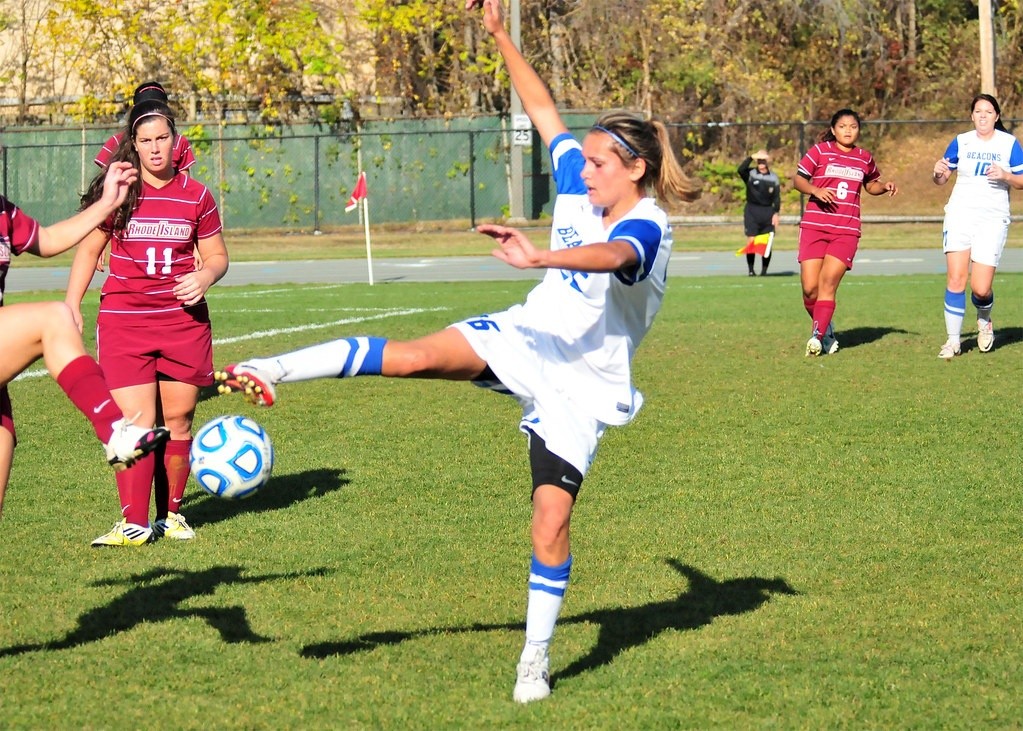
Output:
[748,270,756,276]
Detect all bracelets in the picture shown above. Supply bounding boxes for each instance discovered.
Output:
[936,173,943,178]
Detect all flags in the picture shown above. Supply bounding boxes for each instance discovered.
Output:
[345,173,367,213]
[736,232,773,258]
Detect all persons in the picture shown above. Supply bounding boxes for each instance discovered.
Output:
[0,162,170,510]
[64,79,228,545]
[215,0,704,702]
[933,94,1023,358]
[794,109,899,356]
[737,150,780,276]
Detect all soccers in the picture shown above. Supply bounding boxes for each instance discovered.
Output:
[187,414,274,500]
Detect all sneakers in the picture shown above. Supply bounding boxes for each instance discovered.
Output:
[805,337,821,358]
[102,412,169,473]
[90,516,154,547]
[512,636,553,703]
[823,322,838,354]
[977,313,994,352]
[938,339,962,359]
[215,358,287,407]
[150,512,196,540]
